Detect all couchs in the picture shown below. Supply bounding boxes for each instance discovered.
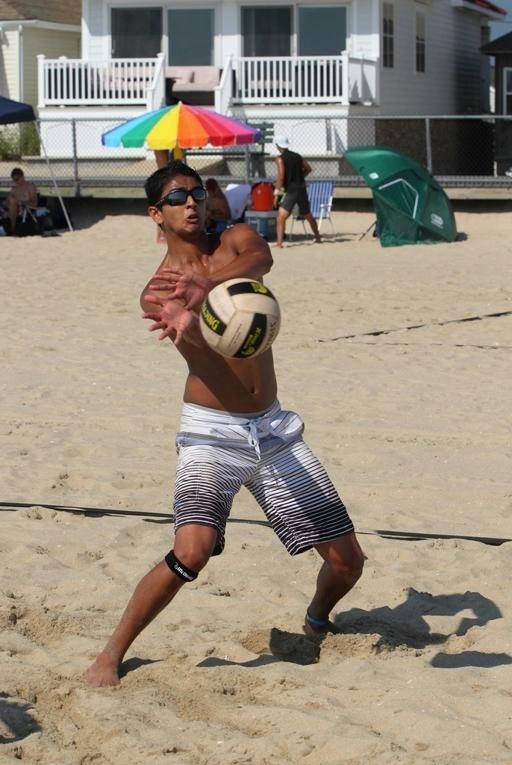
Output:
[100,65,221,103]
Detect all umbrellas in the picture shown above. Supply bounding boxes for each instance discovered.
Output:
[342,142,458,242]
[101,101,264,167]
[0,93,37,129]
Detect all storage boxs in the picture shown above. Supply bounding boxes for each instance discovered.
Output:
[244,210,278,242]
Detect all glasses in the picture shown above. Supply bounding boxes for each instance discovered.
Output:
[155,186,207,207]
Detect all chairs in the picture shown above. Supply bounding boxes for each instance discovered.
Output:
[289,181,335,242]
[224,183,253,223]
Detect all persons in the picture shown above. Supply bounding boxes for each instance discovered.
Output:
[77,156,365,689]
[6,167,41,235]
[205,177,231,230]
[271,135,323,250]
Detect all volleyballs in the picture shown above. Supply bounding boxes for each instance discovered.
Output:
[199,277,280,359]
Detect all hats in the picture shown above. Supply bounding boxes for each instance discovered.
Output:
[275,136,291,149]
